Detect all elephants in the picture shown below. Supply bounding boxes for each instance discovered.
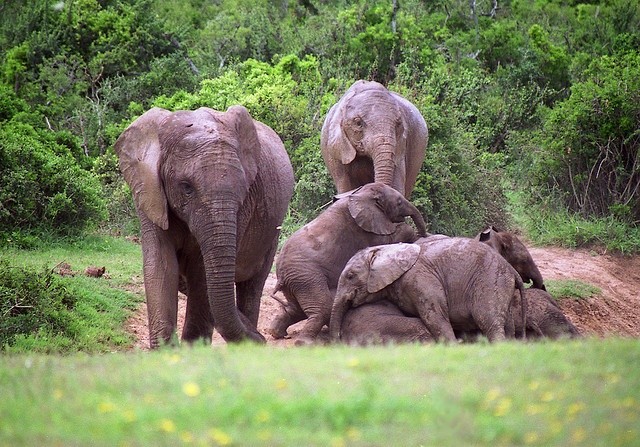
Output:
[321,78,429,201]
[270,180,582,347]
[114,104,295,352]
